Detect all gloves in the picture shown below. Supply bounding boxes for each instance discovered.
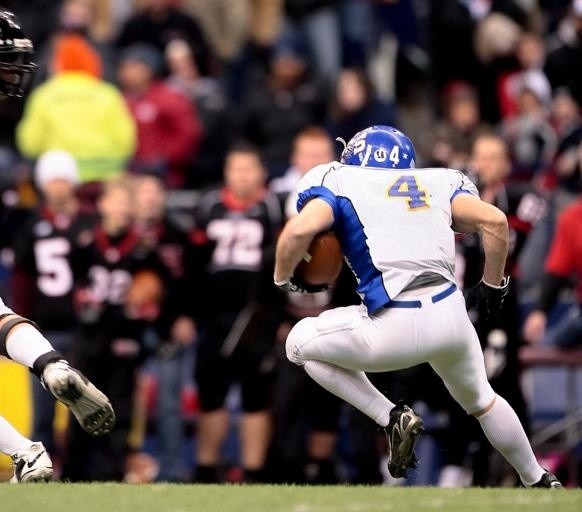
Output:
[272,276,335,294]
[464,276,512,313]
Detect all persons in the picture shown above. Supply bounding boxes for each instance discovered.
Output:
[1,0,581,488]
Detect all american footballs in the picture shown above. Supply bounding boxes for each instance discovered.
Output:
[294,231,344,285]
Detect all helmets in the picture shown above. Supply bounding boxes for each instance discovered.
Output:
[0,6,39,104]
[335,124,418,171]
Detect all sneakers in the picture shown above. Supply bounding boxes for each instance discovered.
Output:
[7,442,54,484]
[39,359,118,438]
[528,469,562,488]
[383,402,425,479]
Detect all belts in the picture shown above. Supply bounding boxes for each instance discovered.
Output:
[381,284,458,309]
